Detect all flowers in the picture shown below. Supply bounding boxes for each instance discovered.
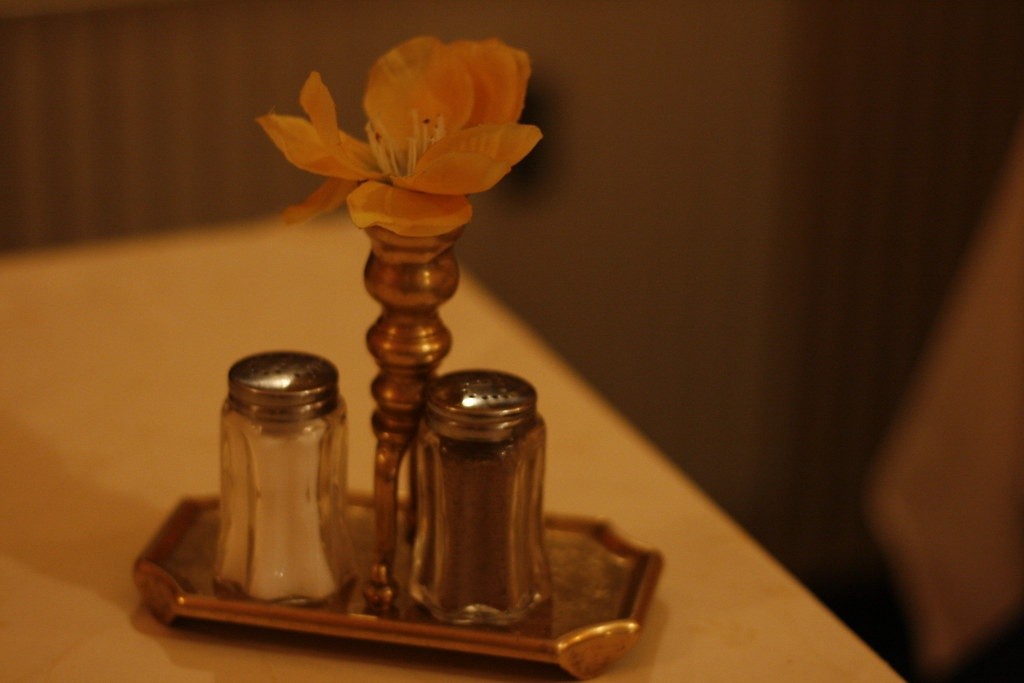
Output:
[253,32,555,236]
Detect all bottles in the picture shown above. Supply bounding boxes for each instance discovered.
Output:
[410,370,549,623]
[213,351,355,605]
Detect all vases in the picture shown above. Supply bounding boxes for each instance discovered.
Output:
[354,246,463,564]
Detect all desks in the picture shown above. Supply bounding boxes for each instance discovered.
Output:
[0,207,910,683]
